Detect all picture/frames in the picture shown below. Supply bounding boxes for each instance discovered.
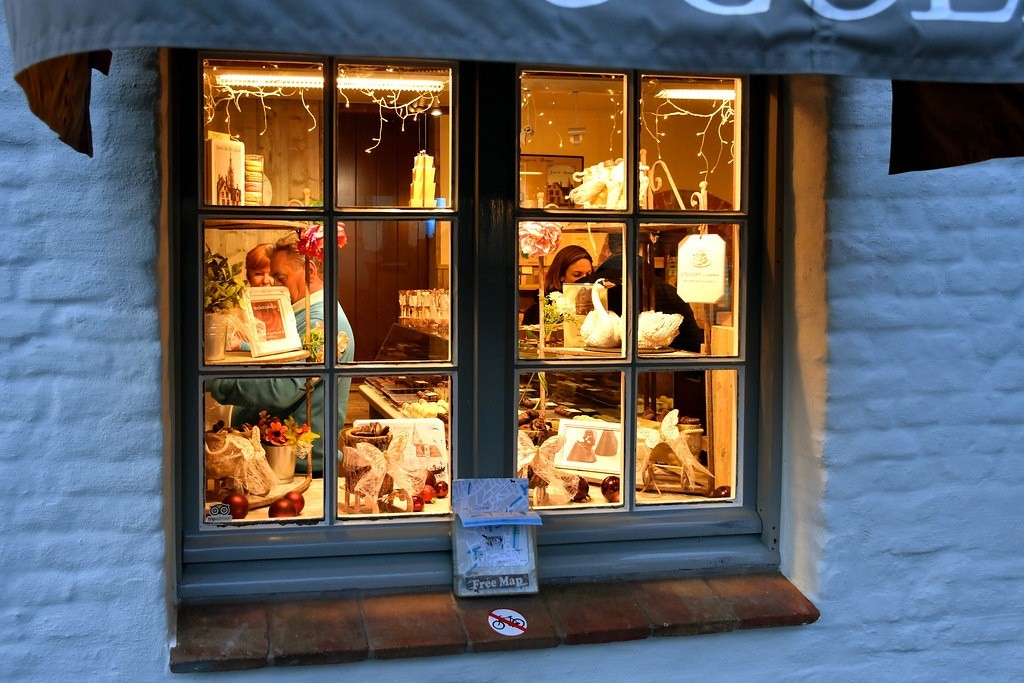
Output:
[520,153,584,209]
[554,417,620,475]
[241,285,301,357]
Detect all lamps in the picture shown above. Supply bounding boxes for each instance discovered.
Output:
[568,95,586,135]
[408,101,415,113]
[430,94,442,116]
[414,97,426,114]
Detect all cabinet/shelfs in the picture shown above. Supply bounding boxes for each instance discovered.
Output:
[537,223,716,497]
[202,219,313,513]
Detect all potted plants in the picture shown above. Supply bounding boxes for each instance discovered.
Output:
[204,245,238,360]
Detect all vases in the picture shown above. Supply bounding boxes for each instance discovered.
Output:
[265,446,295,484]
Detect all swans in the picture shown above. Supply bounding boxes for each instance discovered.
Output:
[581,278,684,347]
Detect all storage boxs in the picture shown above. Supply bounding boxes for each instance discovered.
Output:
[206,139,245,207]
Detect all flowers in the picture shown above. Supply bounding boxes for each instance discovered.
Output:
[254,412,321,446]
[519,220,563,259]
[539,292,577,343]
[297,202,345,261]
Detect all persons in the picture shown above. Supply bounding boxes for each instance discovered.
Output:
[574,233,703,398]
[204,234,354,479]
[522,246,593,325]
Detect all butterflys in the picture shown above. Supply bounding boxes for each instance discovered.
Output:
[514,428,566,482]
[354,431,408,476]
[637,408,680,447]
[227,425,266,461]
